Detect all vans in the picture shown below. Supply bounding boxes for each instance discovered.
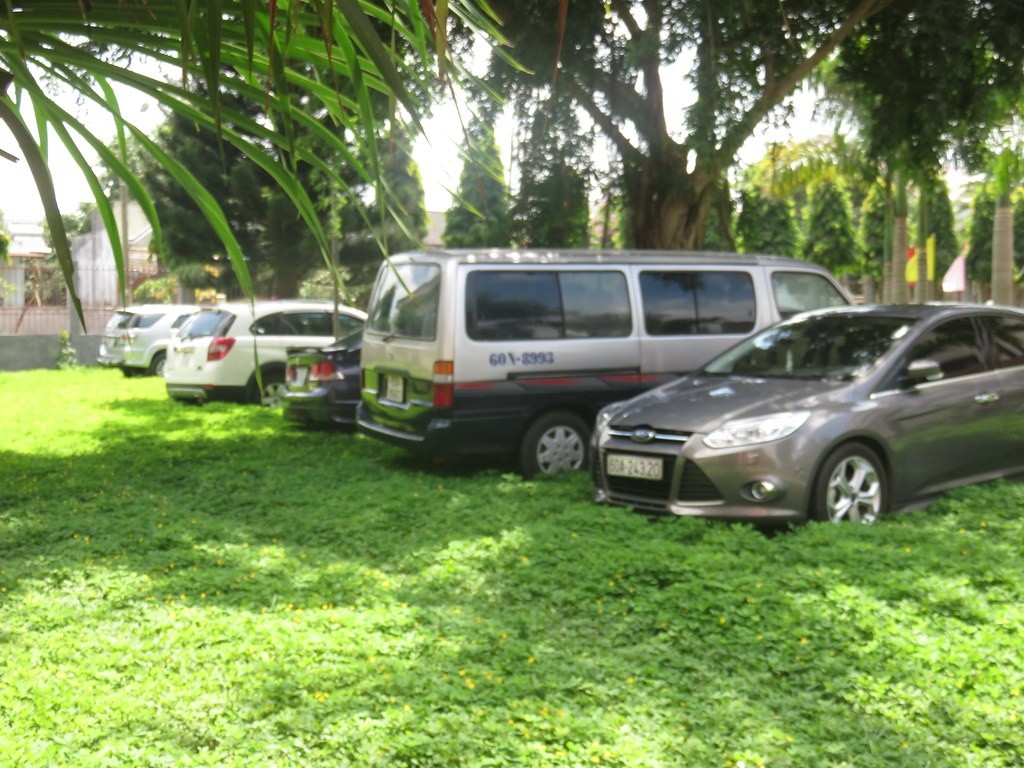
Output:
[354,250,856,480]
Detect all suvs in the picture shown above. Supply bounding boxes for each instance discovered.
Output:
[97,304,200,377]
[164,303,368,407]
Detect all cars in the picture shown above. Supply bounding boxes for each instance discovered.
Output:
[590,302,1024,526]
[281,327,362,429]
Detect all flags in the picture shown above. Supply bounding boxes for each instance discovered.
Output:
[942,253,966,292]
[904,234,934,286]
